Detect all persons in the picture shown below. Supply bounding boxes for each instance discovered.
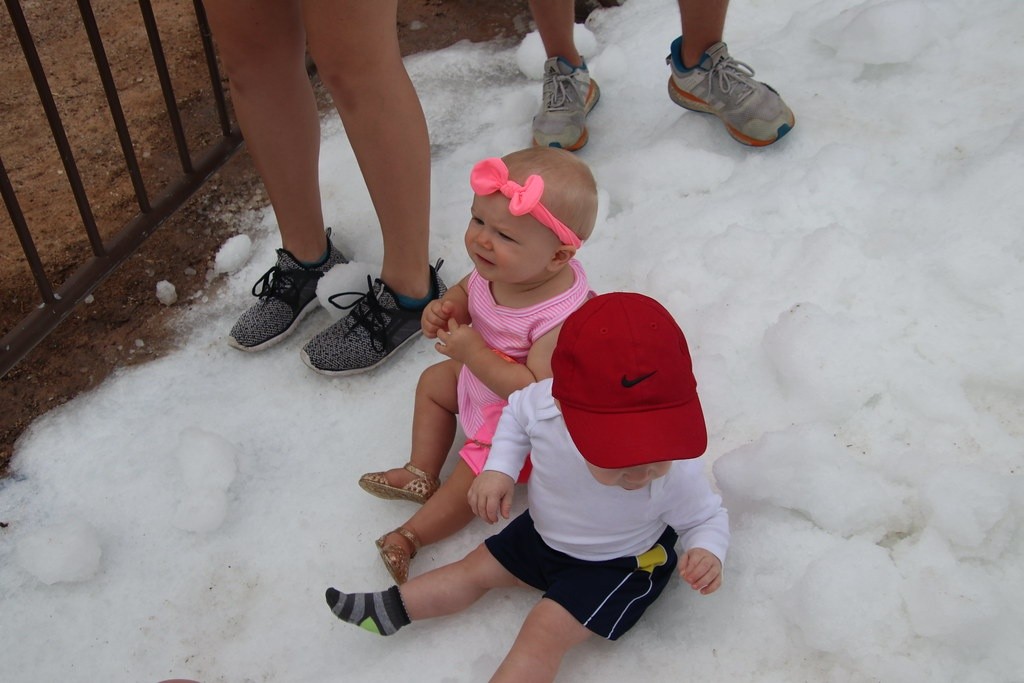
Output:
[326,291,729,683]
[359,147,597,586]
[202,0,448,377]
[527,0,795,152]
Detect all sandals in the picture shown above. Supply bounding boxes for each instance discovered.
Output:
[375,526,420,585]
[359,464,441,504]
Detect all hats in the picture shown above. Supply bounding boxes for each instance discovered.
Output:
[550,292,708,467]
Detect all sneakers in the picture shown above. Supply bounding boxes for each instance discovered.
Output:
[665,34,795,147]
[228,226,348,351]
[300,257,452,375]
[532,55,601,151]
